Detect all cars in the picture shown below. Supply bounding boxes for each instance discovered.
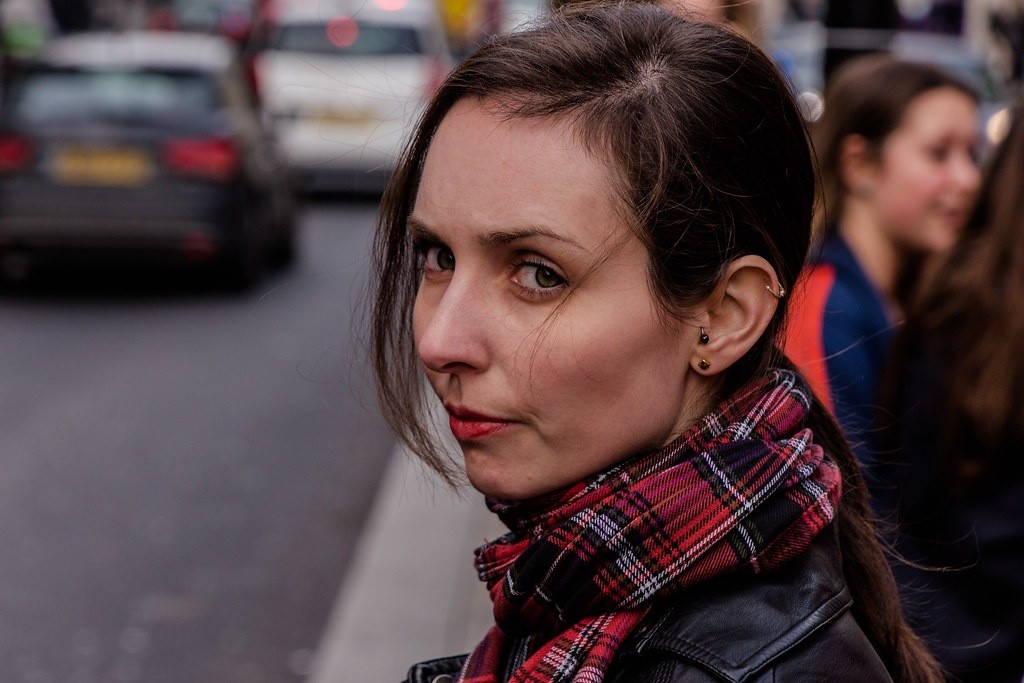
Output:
[247,0,457,201]
[1,27,296,301]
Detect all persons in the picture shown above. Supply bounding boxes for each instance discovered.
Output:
[663,0,1024,680]
[358,0,951,683]
